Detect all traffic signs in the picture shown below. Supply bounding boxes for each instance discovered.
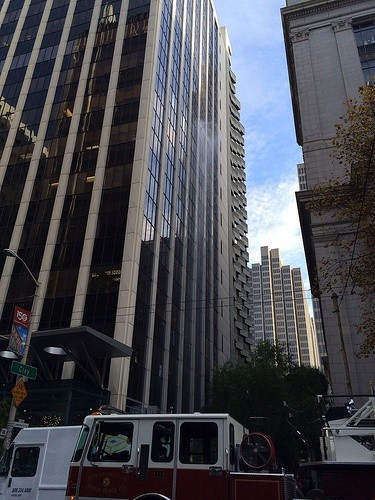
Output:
[9,361,38,380]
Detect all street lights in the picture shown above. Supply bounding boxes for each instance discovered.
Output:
[0,248,42,474]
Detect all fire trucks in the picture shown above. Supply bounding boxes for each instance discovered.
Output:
[1,406,298,499]
